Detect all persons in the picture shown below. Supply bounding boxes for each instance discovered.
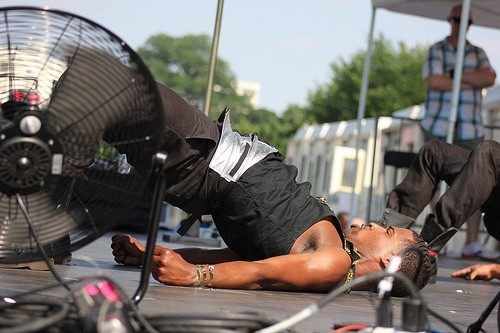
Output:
[422,7,500,261]
[381,136,500,281]
[0,50,439,294]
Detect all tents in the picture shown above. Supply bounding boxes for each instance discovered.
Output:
[356,0,500,126]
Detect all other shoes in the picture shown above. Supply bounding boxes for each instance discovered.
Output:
[0,233,71,271]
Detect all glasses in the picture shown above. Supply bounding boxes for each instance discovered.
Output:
[449,16,472,25]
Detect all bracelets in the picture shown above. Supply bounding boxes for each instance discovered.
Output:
[202,266,207,289]
[194,264,200,288]
[206,265,214,288]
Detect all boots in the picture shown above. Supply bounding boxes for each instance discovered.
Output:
[420,213,458,258]
[378,208,415,229]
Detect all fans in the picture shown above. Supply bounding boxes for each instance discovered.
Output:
[0,6,177,313]
